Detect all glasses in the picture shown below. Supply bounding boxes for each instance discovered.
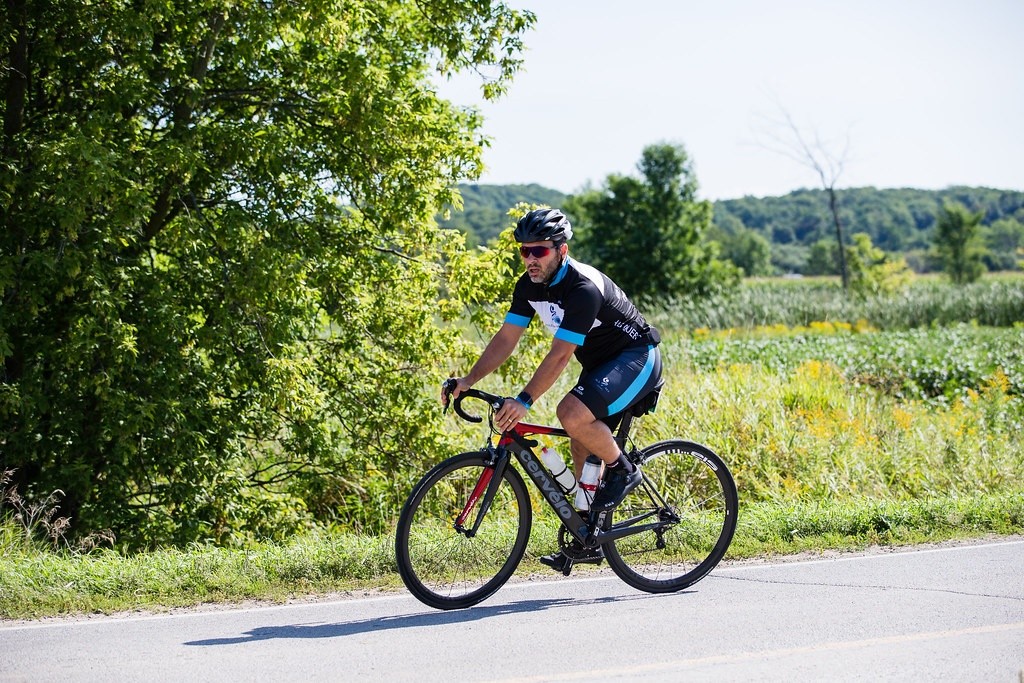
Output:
[518,244,556,258]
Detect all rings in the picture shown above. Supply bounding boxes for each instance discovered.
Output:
[508,419,512,423]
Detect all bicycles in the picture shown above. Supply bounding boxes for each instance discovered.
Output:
[394,378,738,610]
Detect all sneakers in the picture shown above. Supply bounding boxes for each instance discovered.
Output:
[590,462,642,512]
[540,538,604,567]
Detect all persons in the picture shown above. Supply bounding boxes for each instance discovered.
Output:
[441,207,663,572]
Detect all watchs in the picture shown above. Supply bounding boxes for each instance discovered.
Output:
[518,392,533,406]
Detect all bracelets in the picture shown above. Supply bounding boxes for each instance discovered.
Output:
[516,396,530,408]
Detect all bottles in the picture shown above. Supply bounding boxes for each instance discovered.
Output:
[576,452,603,510]
[542,445,578,494]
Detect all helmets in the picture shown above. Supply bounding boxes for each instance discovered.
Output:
[514,206,574,242]
